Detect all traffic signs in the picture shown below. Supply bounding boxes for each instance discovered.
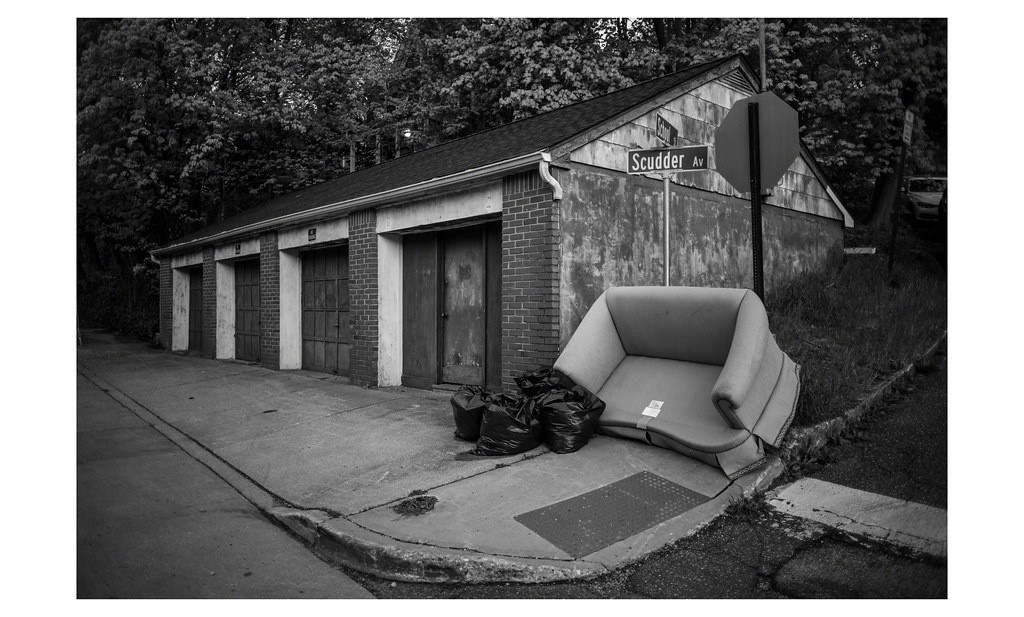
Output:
[626,144,709,174]
[655,112,679,148]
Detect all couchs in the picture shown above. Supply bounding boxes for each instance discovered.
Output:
[554,286,802,481]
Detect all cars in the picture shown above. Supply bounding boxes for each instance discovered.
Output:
[900,175,947,228]
